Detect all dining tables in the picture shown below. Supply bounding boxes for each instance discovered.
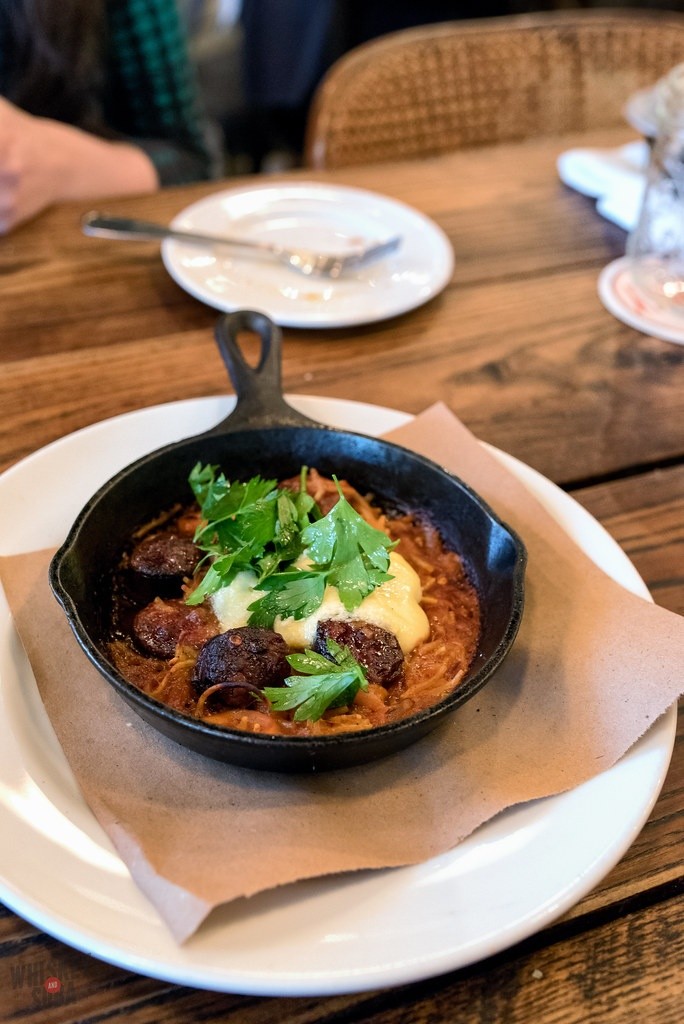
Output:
[0,123,684,1024]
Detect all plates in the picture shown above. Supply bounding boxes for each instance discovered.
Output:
[597,255,682,364]
[1,392,678,999]
[164,174,448,331]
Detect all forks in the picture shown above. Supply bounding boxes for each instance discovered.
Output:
[71,204,406,277]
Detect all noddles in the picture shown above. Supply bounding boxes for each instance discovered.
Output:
[104,462,482,737]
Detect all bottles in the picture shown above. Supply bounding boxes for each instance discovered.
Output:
[616,57,682,323]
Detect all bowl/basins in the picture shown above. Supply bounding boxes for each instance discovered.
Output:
[47,305,527,768]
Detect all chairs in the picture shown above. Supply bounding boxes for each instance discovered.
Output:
[299,6,684,174]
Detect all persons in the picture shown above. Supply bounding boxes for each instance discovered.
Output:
[1,0,333,244]
